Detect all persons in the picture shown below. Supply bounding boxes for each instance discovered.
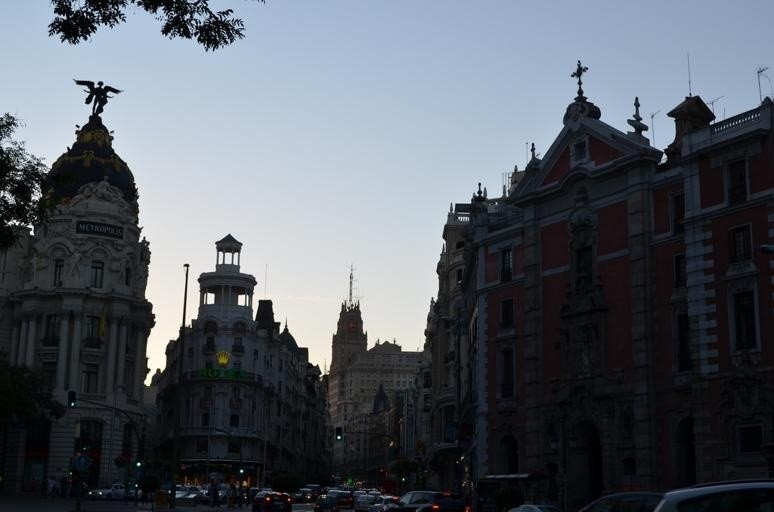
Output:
[29,472,239,511]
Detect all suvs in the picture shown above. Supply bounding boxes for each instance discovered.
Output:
[380,491,469,512]
[655,478,774,511]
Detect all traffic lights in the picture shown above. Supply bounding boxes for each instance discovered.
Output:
[67,390,76,409]
[335,426,343,441]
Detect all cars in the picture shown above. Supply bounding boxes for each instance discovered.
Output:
[578,491,664,512]
[166,482,399,512]
[87,483,126,500]
[126,483,144,500]
[508,504,562,512]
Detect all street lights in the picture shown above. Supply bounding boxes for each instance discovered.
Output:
[168,263,190,510]
[215,428,243,508]
